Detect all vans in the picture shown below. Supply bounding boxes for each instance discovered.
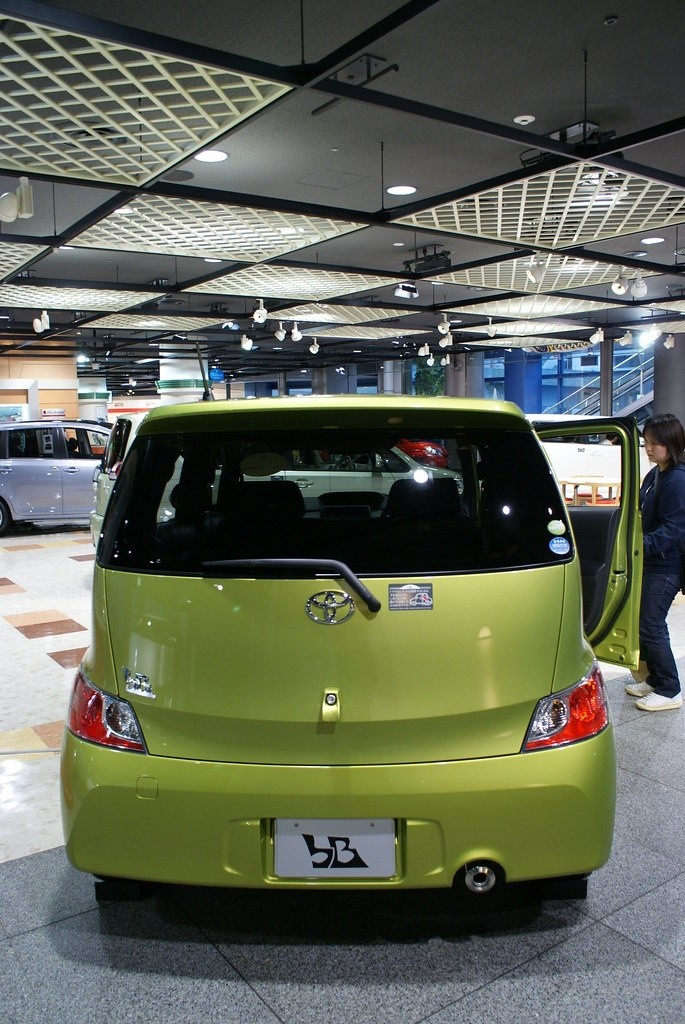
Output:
[524,415,656,500]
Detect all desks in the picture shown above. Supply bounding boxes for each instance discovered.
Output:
[559,477,645,507]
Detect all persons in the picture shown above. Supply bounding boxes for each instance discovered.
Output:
[622,413,685,711]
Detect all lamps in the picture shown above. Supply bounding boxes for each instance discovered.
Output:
[526,251,547,283]
[275,321,287,341]
[417,343,430,356]
[240,334,253,351]
[291,321,303,342]
[589,266,674,349]
[0,177,34,223]
[33,310,50,333]
[309,337,320,355]
[426,353,434,366]
[250,299,268,324]
[437,313,451,335]
[440,354,450,366]
[439,332,453,348]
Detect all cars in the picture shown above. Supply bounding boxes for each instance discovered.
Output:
[61,393,651,912]
[319,437,452,469]
[0,419,169,538]
[238,442,464,504]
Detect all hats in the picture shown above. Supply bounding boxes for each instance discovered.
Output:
[67,437,79,446]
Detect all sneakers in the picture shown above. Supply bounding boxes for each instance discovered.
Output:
[624,681,654,696]
[635,689,682,711]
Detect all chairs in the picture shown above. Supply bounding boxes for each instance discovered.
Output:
[206,481,306,557]
[67,437,80,458]
[375,478,471,573]
